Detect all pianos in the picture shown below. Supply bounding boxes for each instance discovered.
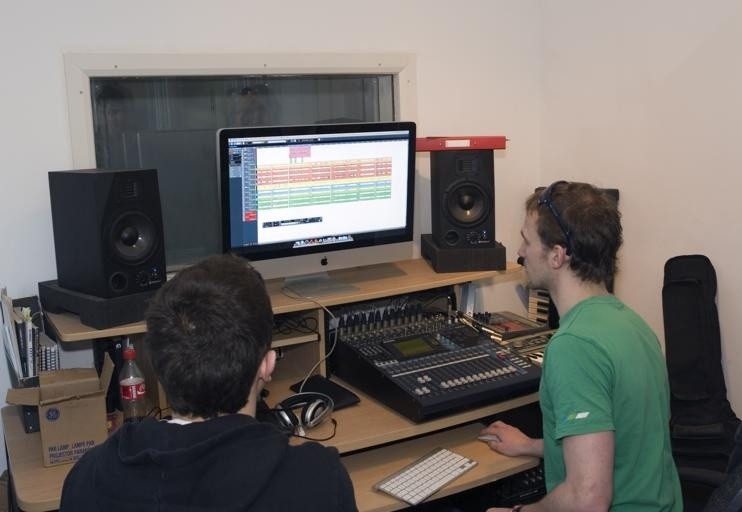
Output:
[529,185,620,334]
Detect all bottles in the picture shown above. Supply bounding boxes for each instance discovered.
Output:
[118,349,146,426]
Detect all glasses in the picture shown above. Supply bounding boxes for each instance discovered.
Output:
[537,181,572,256]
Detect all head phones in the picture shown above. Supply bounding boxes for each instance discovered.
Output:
[273,392,334,432]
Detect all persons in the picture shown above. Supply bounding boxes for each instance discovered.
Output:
[60,253,359,512]
[480,181,686,512]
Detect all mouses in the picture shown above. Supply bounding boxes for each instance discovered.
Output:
[478,434,501,443]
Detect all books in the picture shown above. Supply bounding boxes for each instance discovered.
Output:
[0,284,59,379]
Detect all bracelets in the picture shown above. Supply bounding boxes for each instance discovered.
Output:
[512,504,527,512]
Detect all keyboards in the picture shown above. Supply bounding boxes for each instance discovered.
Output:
[371,446,478,506]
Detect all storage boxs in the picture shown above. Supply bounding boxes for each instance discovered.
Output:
[7,352,117,469]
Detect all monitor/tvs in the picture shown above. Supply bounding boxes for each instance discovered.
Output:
[217,122,416,299]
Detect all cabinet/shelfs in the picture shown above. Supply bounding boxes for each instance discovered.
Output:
[0,256,554,511]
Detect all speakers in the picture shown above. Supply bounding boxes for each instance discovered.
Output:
[430,150,495,249]
[48,169,166,298]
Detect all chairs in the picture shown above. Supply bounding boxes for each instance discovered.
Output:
[676,423,742,512]
